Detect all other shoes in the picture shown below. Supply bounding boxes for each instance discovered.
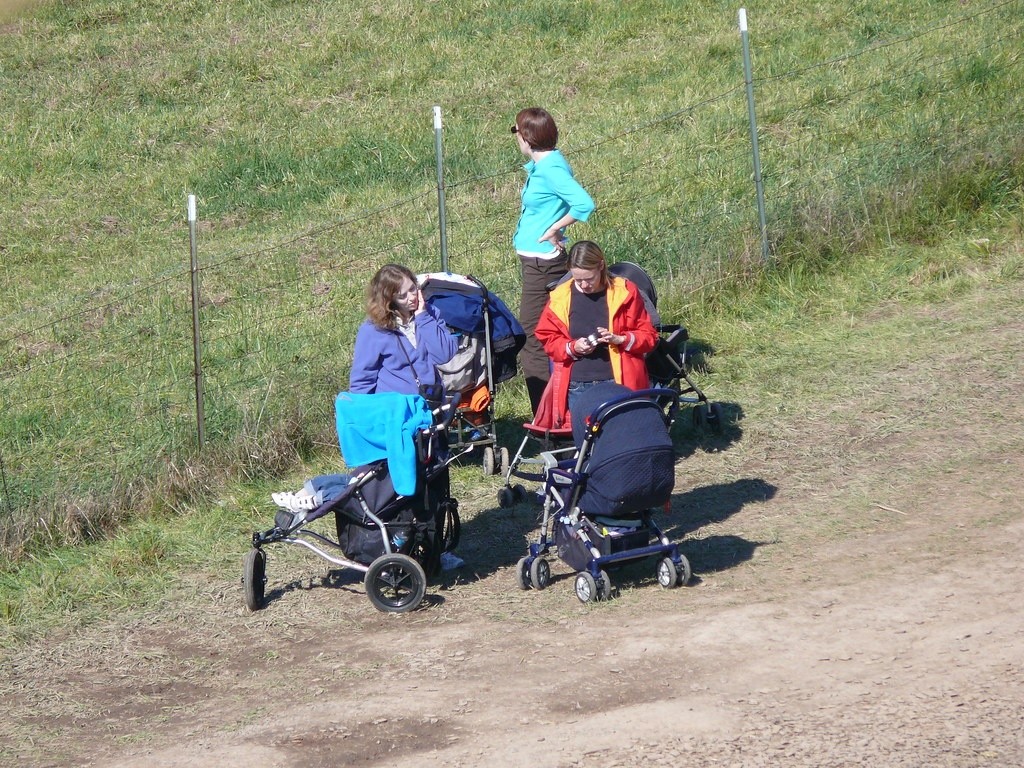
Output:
[271,492,301,514]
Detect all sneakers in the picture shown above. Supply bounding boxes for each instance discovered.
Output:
[439,551,464,571]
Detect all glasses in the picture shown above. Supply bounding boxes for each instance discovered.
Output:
[511,126,519,133]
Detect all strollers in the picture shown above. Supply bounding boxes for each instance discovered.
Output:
[515,382,692,605]
[497,371,579,510]
[240,392,475,614]
[607,261,727,444]
[413,273,509,478]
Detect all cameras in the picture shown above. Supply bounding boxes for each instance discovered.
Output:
[584,333,599,347]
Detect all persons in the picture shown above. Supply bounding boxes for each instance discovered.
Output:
[271,457,396,521]
[351,265,464,572]
[511,107,595,419]
[533,241,661,412]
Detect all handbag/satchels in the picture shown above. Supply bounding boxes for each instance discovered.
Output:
[418,383,444,426]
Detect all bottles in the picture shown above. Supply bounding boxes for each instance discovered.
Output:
[381,526,411,556]
